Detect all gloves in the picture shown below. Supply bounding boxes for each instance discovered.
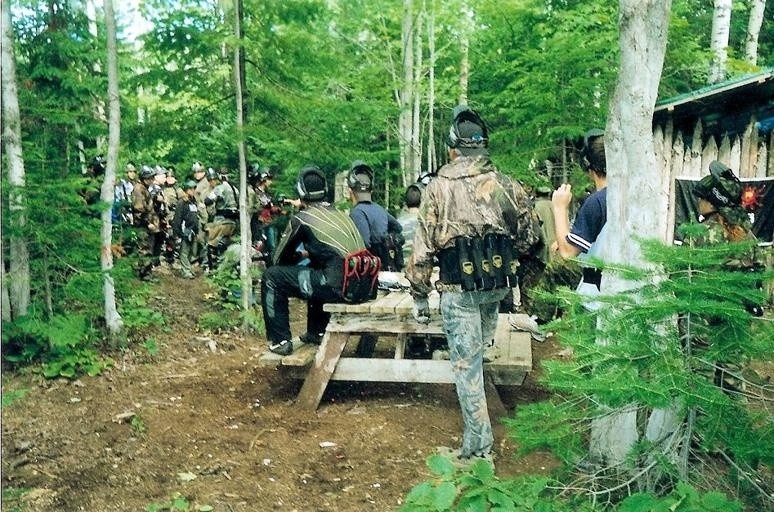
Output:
[412,297,430,324]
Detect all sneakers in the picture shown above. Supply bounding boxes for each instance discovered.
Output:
[508,314,548,343]
[298,333,323,345]
[435,446,496,471]
[267,340,293,355]
[139,260,198,283]
[483,345,500,363]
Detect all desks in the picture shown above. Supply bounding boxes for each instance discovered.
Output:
[296,292,511,420]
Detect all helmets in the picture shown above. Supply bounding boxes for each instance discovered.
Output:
[125,161,220,190]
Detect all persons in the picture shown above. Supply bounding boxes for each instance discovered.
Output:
[73,152,301,283]
[257,155,597,356]
[403,103,544,475]
[548,128,607,431]
[668,159,763,468]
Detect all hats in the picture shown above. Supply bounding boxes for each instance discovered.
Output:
[692,160,744,207]
[345,164,375,192]
[445,109,489,148]
[294,167,328,200]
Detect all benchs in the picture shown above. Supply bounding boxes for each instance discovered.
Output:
[485,314,533,386]
[258,334,322,380]
